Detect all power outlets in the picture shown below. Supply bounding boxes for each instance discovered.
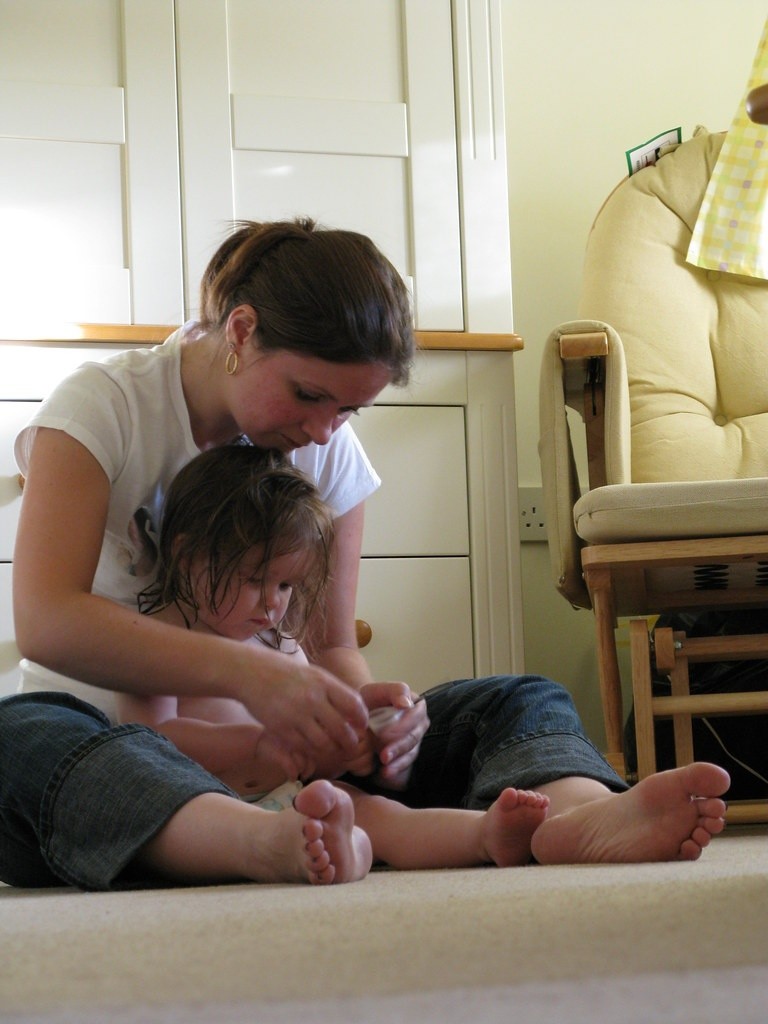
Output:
[518,485,549,540]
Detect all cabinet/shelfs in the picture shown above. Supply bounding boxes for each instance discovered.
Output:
[0,1,465,333]
[0,344,522,709]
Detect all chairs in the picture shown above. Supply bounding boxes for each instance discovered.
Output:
[559,133,768,823]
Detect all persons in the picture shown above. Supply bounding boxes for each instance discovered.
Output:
[1,217,732,884]
